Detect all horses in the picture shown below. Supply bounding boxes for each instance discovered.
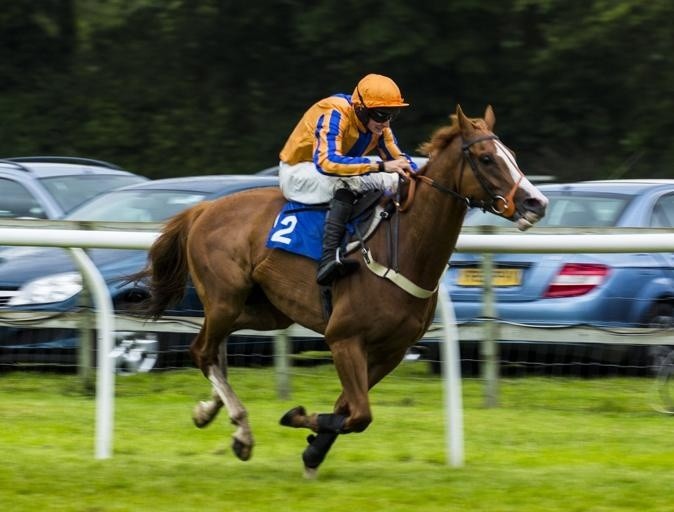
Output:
[109,103,550,481]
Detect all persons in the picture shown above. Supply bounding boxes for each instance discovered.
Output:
[276,71,421,287]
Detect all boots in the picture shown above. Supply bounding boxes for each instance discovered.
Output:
[313,197,366,288]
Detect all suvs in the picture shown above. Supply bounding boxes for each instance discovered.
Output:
[0,154,149,223]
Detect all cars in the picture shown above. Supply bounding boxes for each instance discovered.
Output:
[416,178,674,376]
[1,175,315,375]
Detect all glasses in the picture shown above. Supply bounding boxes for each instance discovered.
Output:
[367,110,399,123]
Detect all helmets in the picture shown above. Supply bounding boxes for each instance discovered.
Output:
[350,73,409,108]
